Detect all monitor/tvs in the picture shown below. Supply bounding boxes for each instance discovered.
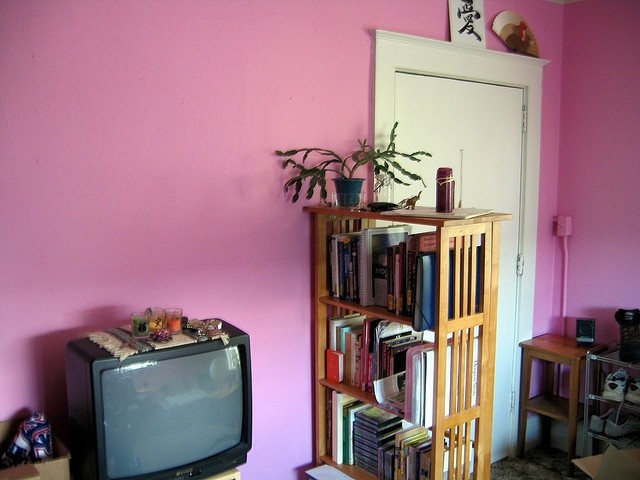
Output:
[64,316,253,480]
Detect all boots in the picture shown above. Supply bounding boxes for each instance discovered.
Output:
[614,308,640,363]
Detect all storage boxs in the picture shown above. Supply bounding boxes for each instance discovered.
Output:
[0,415,71,480]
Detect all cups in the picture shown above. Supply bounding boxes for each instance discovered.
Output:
[146,307,164,335]
[131,312,149,338]
[166,308,183,334]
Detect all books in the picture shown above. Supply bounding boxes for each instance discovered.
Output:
[331,390,472,480]
[330,226,483,331]
[326,313,480,428]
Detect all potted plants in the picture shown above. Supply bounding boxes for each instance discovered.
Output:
[275,122,433,209]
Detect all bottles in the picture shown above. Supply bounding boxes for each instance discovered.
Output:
[437,168,455,212]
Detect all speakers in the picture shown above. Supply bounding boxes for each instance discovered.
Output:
[576,318,596,349]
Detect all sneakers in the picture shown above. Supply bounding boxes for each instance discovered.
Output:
[602,367,631,402]
[603,411,640,439]
[625,375,640,405]
[588,407,630,435]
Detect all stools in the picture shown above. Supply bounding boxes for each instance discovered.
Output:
[518,333,608,477]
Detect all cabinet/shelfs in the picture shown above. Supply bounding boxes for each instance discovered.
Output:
[581,340,640,456]
[302,206,514,476]
